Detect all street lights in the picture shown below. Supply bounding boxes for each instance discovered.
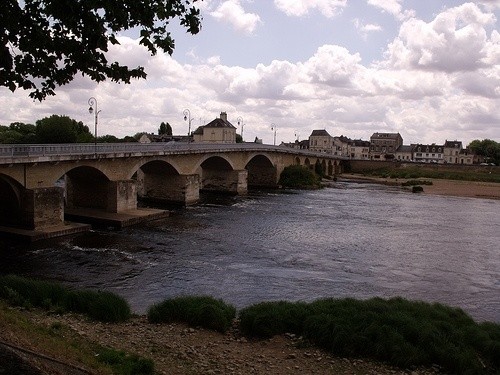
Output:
[270,123,277,145]
[88,97,101,145]
[182,109,193,151]
[237,116,245,143]
